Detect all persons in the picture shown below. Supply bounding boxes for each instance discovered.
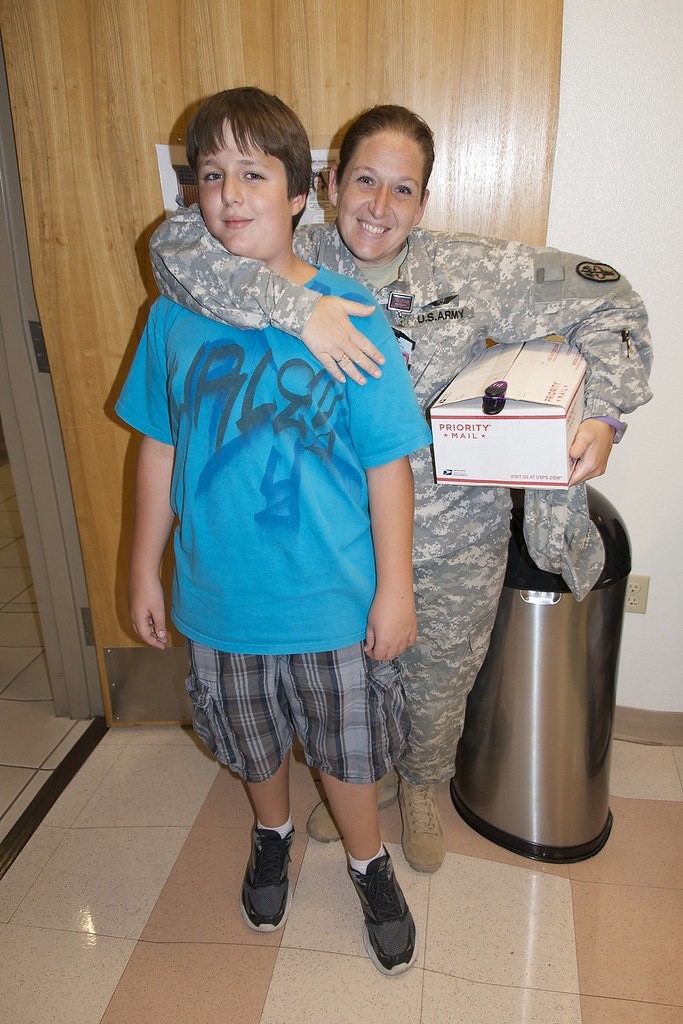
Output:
[112,85,434,975]
[146,102,654,873]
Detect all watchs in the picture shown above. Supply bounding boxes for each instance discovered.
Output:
[601,413,628,445]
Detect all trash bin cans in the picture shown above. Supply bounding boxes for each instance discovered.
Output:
[441,472,640,865]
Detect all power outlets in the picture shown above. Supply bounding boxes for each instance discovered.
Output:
[624,573,650,613]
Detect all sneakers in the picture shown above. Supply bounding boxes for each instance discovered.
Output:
[238,816,296,933]
[306,768,399,844]
[348,846,420,975]
[397,776,446,873]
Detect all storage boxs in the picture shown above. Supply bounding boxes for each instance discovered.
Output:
[427,335,587,491]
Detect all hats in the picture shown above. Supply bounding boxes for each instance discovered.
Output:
[522,481,605,603]
